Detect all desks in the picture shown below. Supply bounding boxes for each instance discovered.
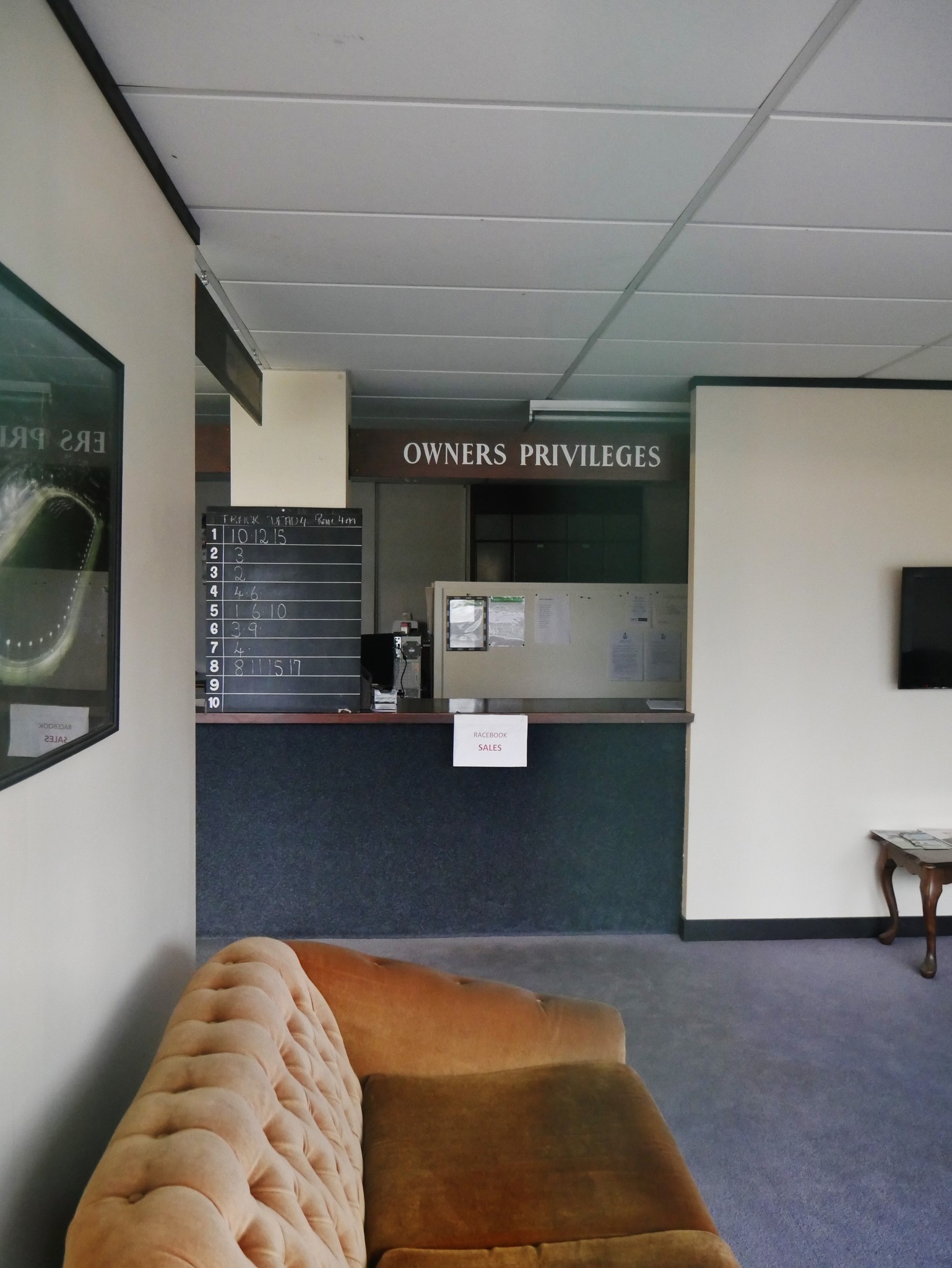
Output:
[870,829,952,979]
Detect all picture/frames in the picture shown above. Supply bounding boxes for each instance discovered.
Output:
[1,258,123,789]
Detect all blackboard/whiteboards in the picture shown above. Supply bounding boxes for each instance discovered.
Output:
[205,503,364,712]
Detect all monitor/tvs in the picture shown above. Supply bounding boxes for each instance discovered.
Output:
[899,566,952,689]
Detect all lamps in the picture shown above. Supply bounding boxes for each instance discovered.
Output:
[528,399,692,424]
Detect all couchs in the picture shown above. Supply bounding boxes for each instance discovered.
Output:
[60,936,745,1268]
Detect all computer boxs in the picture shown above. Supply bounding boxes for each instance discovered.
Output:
[394,636,422,698]
